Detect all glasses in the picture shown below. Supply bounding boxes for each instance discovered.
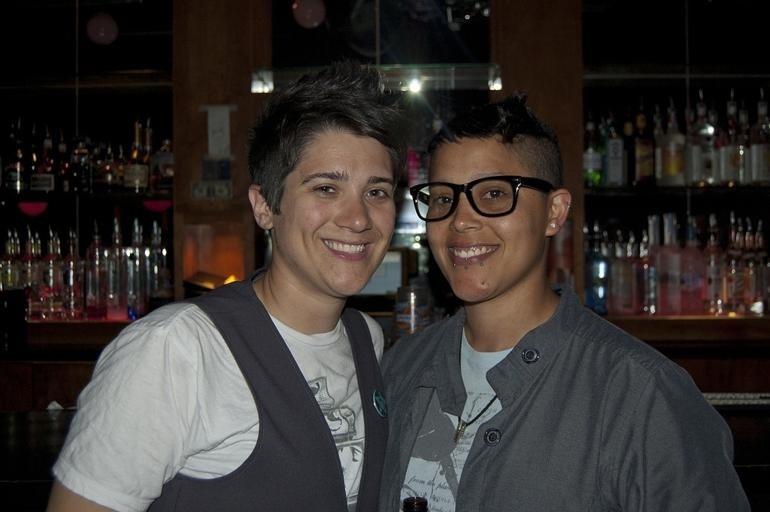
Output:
[409,175,555,223]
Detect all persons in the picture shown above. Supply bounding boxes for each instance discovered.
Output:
[377,95,752,512]
[42,60,411,512]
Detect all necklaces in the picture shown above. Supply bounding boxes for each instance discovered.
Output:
[454,393,498,443]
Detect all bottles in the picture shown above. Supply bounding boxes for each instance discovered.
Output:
[0,119,170,321]
[402,497,430,512]
[585,86,770,319]
[396,277,429,335]
